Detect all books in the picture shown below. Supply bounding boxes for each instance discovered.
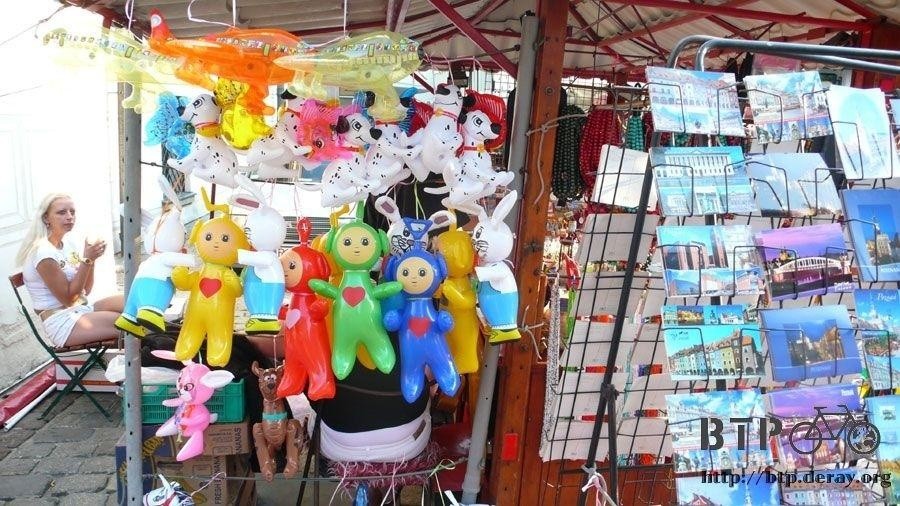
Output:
[537,64,900,506]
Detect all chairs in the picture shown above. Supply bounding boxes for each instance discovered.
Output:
[292,376,437,505]
[7,272,117,424]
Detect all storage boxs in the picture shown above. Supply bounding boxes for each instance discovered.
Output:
[55,348,124,392]
[115,415,250,505]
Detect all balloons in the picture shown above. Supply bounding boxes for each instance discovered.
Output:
[39,7,522,483]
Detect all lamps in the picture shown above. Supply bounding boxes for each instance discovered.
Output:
[447,69,468,87]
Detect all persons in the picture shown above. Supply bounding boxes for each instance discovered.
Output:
[15,194,124,349]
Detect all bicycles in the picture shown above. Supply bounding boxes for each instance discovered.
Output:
[790,402,880,454]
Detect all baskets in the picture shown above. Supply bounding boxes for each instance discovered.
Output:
[122,380,244,426]
